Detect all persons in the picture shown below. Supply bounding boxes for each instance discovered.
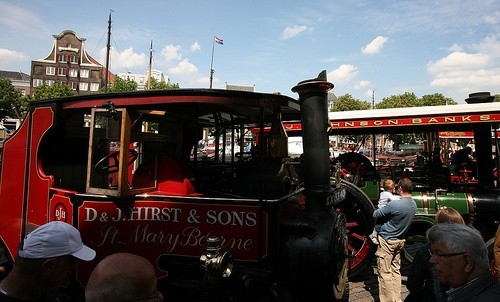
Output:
[427,222,500,302]
[370,180,400,245]
[0,220,96,302]
[375,178,417,302]
[85,253,163,302]
[404,208,464,302]
[492,224,500,283]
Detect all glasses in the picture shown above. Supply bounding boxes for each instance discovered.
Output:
[428,245,464,263]
[127,287,158,301]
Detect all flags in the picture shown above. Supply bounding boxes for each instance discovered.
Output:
[214,36,224,45]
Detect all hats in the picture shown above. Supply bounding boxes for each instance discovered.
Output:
[19,220,97,262]
